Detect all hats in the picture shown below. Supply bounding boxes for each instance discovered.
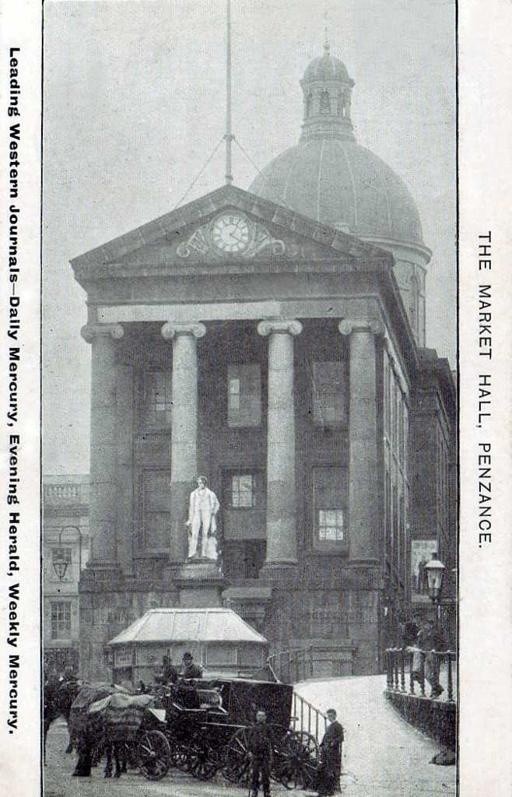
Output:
[183,653,194,660]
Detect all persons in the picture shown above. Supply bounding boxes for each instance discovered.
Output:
[248,709,278,797]
[183,474,221,561]
[417,616,446,700]
[405,610,426,683]
[314,709,345,797]
[160,654,177,687]
[138,679,145,695]
[181,651,204,680]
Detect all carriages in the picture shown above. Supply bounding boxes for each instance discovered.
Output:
[42,667,322,792]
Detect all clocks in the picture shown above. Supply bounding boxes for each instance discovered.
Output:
[207,212,255,259]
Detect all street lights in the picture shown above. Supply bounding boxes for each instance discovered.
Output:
[425,547,447,654]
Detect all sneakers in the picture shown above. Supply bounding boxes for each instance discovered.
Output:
[430,685,444,696]
[252,792,271,797]
